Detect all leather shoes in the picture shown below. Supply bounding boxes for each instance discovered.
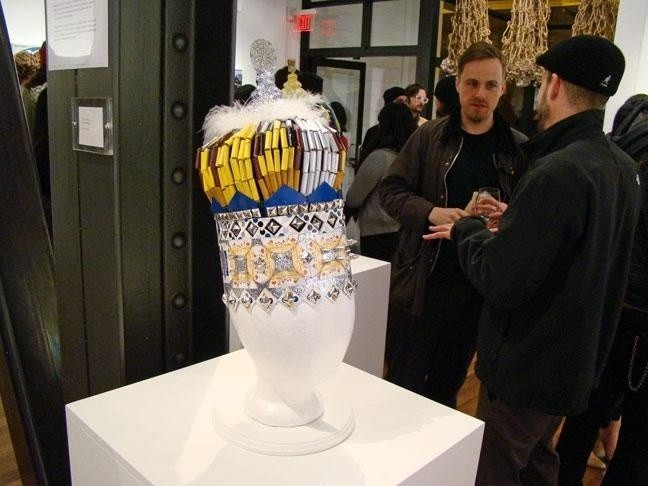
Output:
[552,431,612,471]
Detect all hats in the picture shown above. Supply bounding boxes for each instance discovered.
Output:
[535,34,627,97]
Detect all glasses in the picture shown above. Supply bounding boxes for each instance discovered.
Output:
[414,94,430,103]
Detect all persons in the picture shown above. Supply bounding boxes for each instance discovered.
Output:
[15,44,49,162]
[236,58,428,261]
[379,36,647,486]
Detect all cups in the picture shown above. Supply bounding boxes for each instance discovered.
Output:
[474,188,501,229]
